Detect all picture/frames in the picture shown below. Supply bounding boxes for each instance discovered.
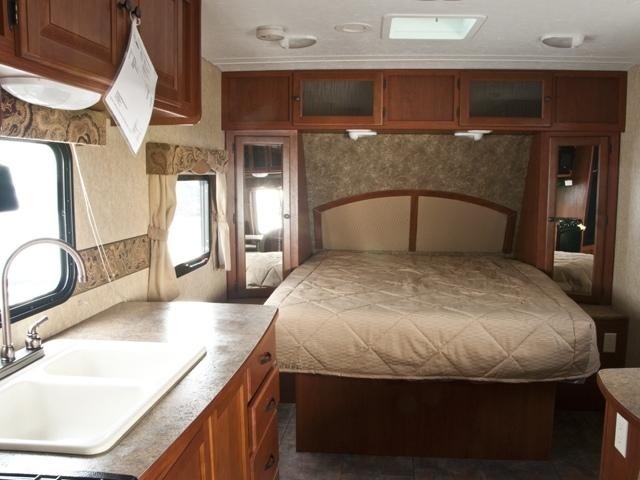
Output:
[595,368,640,480]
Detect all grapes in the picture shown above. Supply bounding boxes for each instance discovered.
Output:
[578,305,629,383]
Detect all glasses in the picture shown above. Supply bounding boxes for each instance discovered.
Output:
[0,76,101,111]
[257,25,285,42]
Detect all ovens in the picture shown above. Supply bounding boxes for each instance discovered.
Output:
[265,187,601,461]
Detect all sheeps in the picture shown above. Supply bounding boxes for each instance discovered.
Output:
[1,238,88,363]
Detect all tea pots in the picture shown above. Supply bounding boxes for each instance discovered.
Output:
[553,143,600,295]
[243,141,283,291]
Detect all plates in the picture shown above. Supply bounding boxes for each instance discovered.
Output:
[516,134,620,306]
[0,1,202,125]
[247,309,281,480]
[139,360,249,480]
[226,129,311,300]
